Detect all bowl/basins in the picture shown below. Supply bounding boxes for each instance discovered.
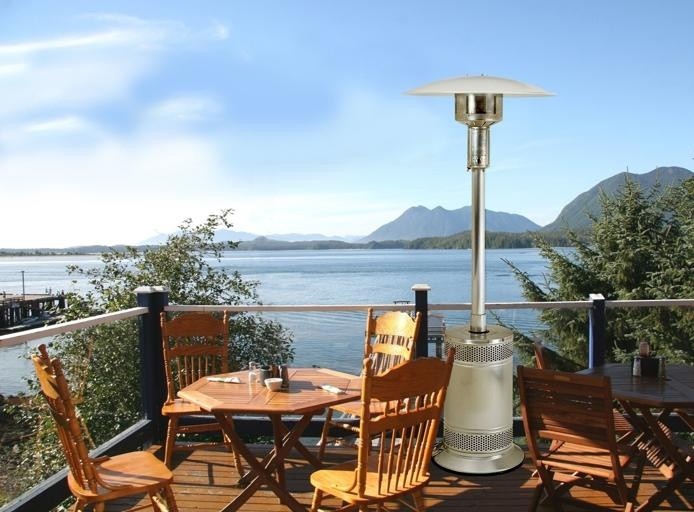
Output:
[264,378,283,391]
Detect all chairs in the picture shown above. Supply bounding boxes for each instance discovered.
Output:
[155,308,248,481]
[28,342,181,511]
[314,303,423,462]
[527,336,636,484]
[307,346,458,512]
[509,364,648,512]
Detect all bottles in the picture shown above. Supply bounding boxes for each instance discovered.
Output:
[630,355,666,378]
[249,362,289,387]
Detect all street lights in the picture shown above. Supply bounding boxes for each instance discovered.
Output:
[18,271,28,301]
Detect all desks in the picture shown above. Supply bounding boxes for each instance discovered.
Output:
[174,363,365,511]
[569,359,694,512]
